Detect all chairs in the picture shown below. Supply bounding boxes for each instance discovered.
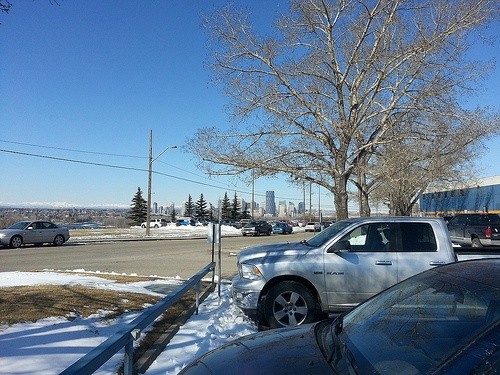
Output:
[372,229,392,250]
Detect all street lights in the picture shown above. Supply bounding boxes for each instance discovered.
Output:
[146,146,177,236]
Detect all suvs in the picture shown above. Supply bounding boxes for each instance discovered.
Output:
[445,213,500,247]
[141,218,167,229]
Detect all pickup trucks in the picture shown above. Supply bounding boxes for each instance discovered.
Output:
[271,222,293,235]
[230,217,500,331]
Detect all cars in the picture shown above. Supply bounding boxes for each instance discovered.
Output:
[176,216,210,227]
[175,256,500,375]
[0,219,70,249]
[305,222,321,232]
[222,217,336,230]
[241,220,272,237]
